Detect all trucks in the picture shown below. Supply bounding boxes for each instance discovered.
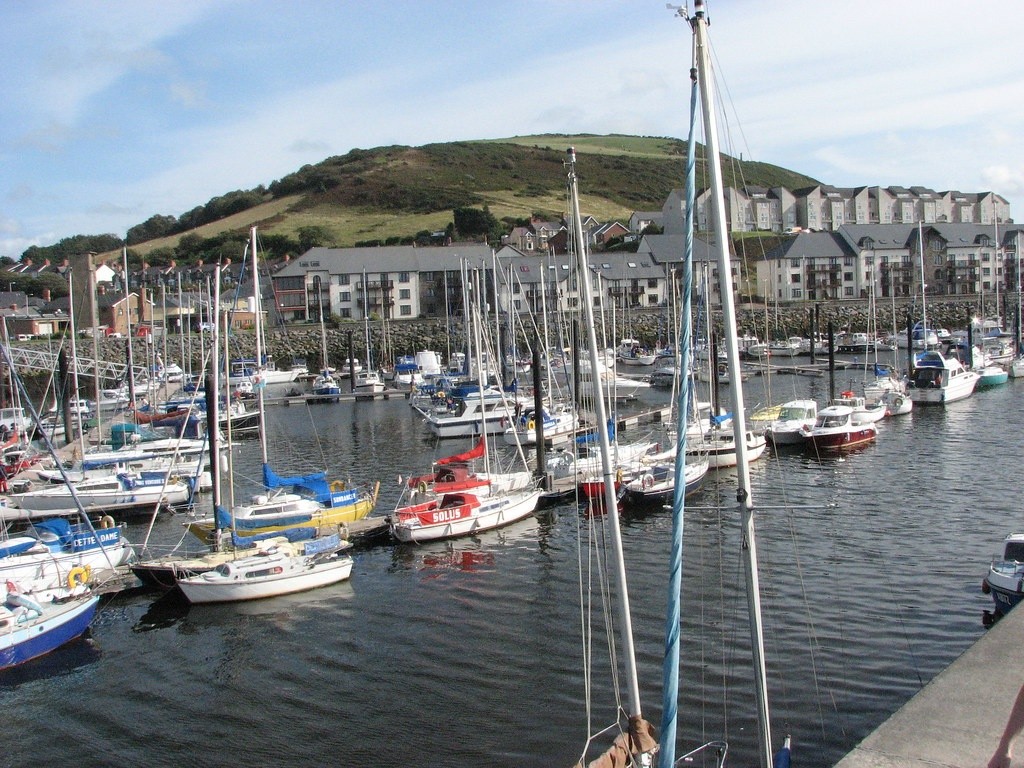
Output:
[132,324,169,338]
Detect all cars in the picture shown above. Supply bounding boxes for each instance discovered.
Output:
[15,333,27,342]
[27,333,34,340]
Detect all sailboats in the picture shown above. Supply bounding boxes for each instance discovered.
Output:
[0,224,1023,678]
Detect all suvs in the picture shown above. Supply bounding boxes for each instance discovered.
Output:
[193,321,216,335]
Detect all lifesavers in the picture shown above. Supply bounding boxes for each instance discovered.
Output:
[68,568,88,588]
[100,516,116,529]
[527,420,535,429]
[418,481,427,495]
[641,474,655,489]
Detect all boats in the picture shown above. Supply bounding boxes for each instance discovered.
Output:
[983,533,1022,616]
